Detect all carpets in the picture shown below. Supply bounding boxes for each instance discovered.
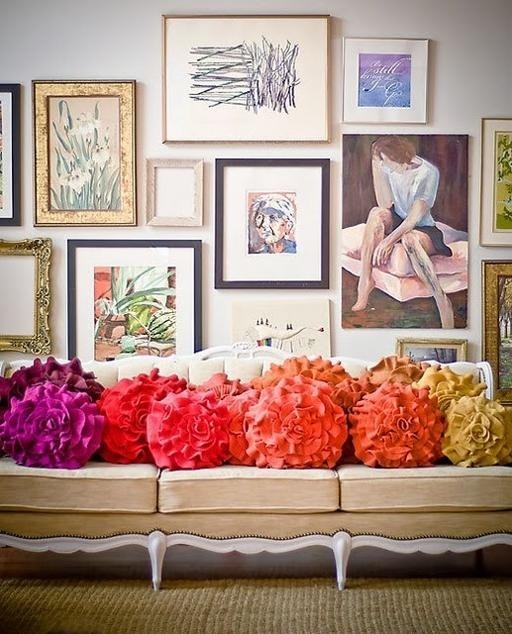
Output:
[1,577,512,634]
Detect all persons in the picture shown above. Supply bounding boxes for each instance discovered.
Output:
[248,193,295,253]
[350,136,455,329]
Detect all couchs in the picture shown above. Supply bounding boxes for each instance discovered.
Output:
[0,342,512,591]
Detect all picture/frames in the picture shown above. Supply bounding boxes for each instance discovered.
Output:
[482,259,512,405]
[227,297,332,357]
[396,338,468,366]
[0,82,22,226]
[144,156,206,228]
[161,14,332,145]
[0,237,52,357]
[32,80,137,227]
[214,158,331,289]
[68,239,202,360]
[340,133,472,329]
[481,117,512,247]
[341,36,430,126]
